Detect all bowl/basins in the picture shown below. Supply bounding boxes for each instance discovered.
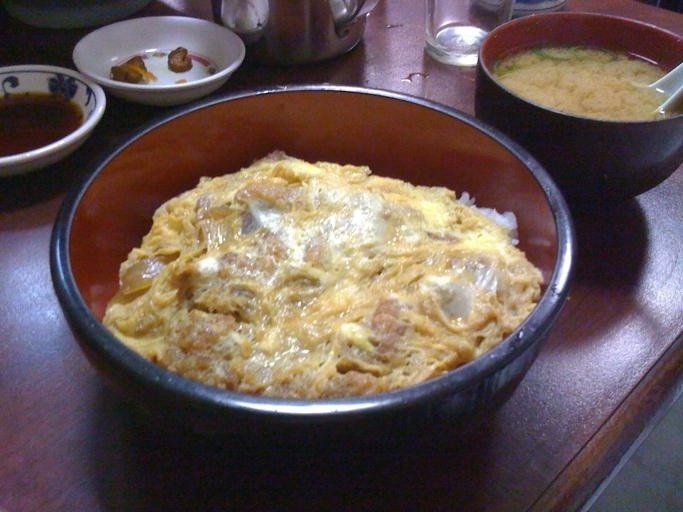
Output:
[0,64,108,183]
[47,81,580,471]
[475,9,682,213]
[70,12,247,110]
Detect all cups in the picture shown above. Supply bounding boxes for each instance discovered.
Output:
[210,0,381,75]
[421,0,565,71]
